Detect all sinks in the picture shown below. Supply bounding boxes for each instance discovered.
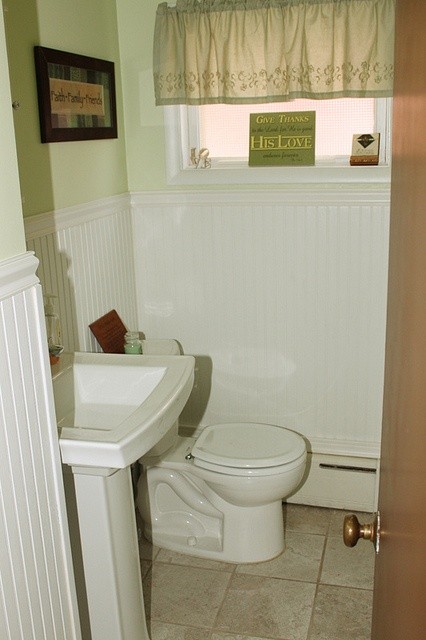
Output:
[47,351,195,468]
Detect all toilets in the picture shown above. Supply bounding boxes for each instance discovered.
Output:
[136,338,308,563]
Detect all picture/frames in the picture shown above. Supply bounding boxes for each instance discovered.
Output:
[32,45,118,144]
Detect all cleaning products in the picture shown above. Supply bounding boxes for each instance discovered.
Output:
[123,333,142,354]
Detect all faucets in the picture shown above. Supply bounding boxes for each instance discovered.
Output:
[48,344,65,356]
